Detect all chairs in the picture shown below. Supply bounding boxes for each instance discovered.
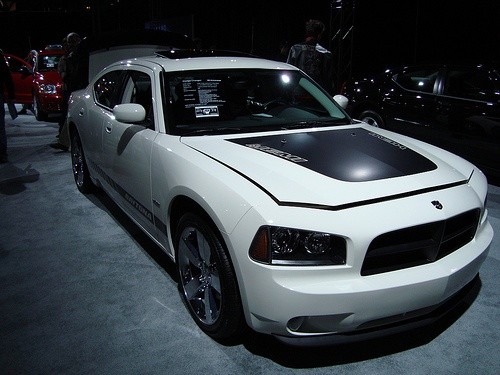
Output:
[229,73,279,115]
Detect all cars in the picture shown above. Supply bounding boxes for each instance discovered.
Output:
[68,45,494,337]
[338,41,500,176]
[3,49,66,119]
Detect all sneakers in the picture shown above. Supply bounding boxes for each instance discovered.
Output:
[50,143,69,152]
[17,108,27,115]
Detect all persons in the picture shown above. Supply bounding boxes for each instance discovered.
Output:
[287,20,333,108]
[49,33,95,152]
[17,50,38,115]
[55,37,70,137]
[0,47,16,167]
